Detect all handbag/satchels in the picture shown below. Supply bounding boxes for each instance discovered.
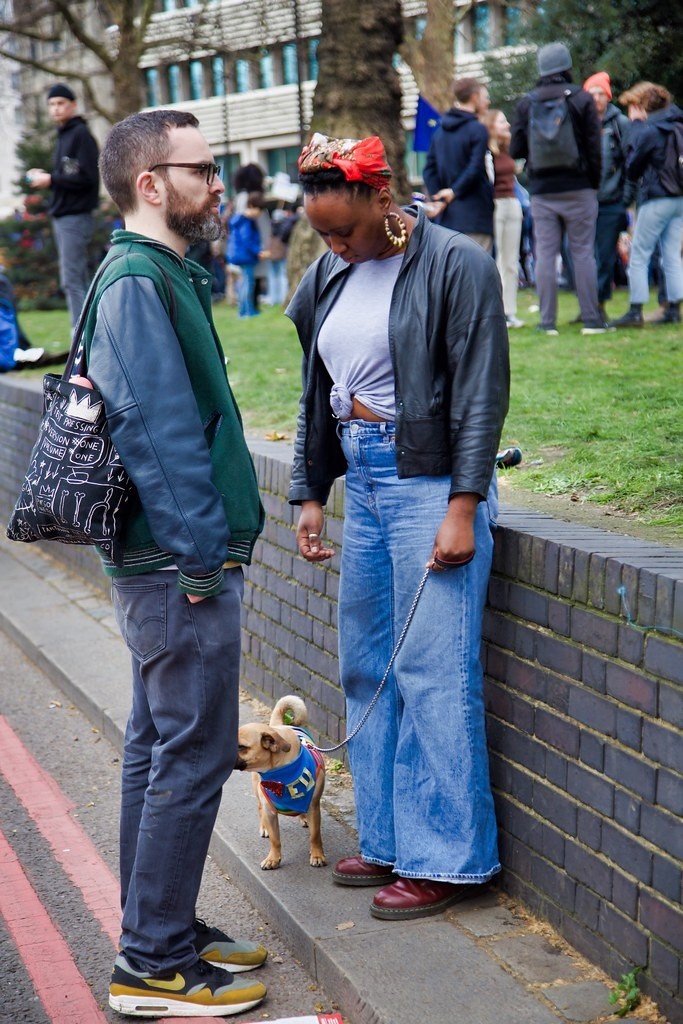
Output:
[0,298,20,371]
[6,252,177,569]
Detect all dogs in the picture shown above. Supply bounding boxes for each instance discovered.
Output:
[234,694,327,870]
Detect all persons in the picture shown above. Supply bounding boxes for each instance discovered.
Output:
[71,111,268,1017]
[0,264,30,375]
[514,175,660,289]
[509,40,617,335]
[611,81,683,329]
[423,76,525,329]
[24,83,100,346]
[568,72,635,323]
[185,165,306,319]
[283,132,511,920]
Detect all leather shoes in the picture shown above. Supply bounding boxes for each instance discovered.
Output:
[331,855,399,885]
[613,311,644,326]
[370,876,488,920]
[653,312,680,323]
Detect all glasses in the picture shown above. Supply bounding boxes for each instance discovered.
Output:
[148,162,221,184]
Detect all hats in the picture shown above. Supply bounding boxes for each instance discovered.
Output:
[583,71,613,99]
[537,43,571,76]
[45,84,75,101]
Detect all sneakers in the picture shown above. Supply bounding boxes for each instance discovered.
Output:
[107,954,267,1017]
[117,916,266,972]
[535,325,559,335]
[581,319,617,333]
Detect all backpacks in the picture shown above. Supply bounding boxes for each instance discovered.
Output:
[659,121,683,195]
[527,85,581,170]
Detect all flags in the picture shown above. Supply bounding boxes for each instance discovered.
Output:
[414,95,443,152]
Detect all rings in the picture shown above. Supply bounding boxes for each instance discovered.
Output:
[309,534,319,537]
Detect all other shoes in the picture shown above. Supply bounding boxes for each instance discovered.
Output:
[506,316,526,328]
[570,311,609,322]
